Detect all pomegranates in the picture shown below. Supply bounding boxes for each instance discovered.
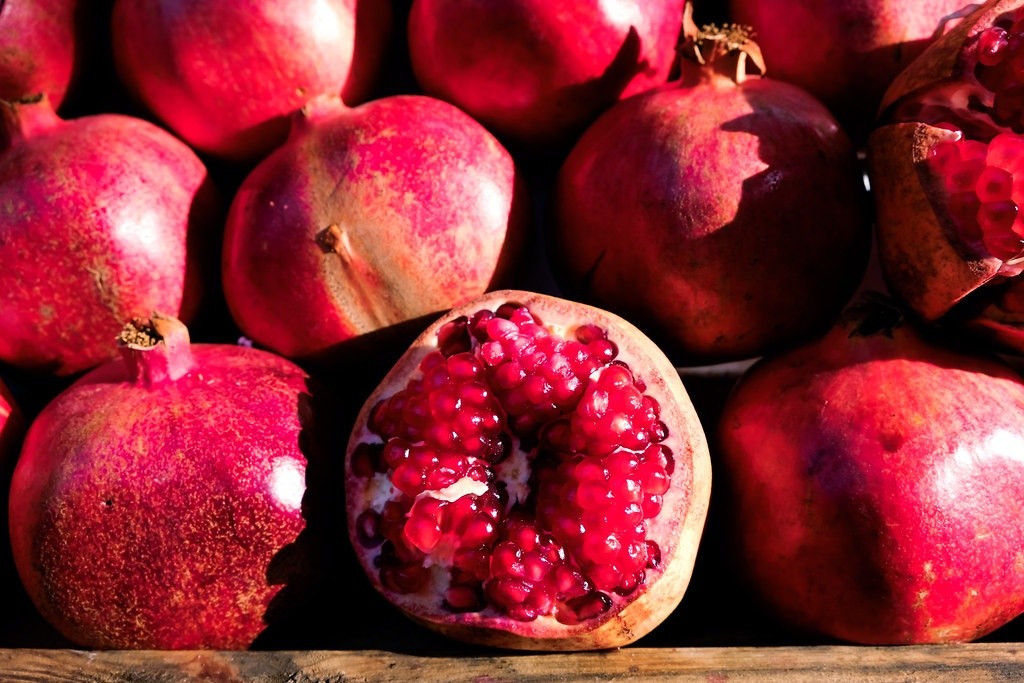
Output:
[1,1,1024,649]
